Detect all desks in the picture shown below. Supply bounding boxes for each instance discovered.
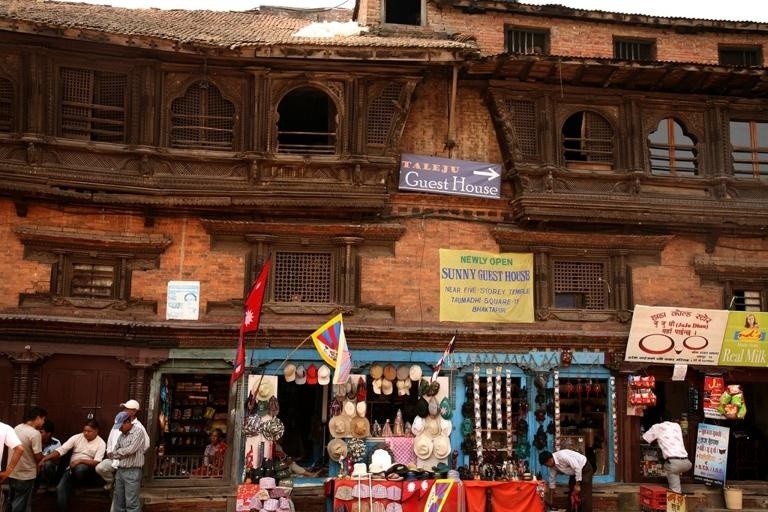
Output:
[331,472,546,512]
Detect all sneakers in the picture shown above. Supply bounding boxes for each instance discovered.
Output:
[104,482,113,490]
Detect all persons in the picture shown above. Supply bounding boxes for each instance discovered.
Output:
[190,429,228,479]
[94,399,150,491]
[538,447,593,512]
[109,411,146,512]
[31,418,61,512]
[273,439,322,478]
[0,421,25,512]
[5,406,47,512]
[639,405,692,496]
[738,313,761,343]
[34,417,106,512]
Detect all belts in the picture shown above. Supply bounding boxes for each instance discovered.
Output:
[666,456,689,464]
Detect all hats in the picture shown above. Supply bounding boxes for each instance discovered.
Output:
[254,378,274,401]
[416,379,451,419]
[329,377,370,438]
[119,399,140,410]
[352,464,372,477]
[369,449,391,473]
[257,396,279,416]
[328,439,347,462]
[261,417,285,440]
[336,484,403,512]
[372,462,450,481]
[411,416,453,460]
[249,477,290,511]
[345,438,367,463]
[284,363,331,385]
[370,364,422,395]
[113,412,129,429]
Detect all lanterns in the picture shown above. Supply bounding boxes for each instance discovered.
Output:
[575,383,583,400]
[585,383,591,400]
[592,382,602,400]
[565,382,572,399]
[560,346,572,369]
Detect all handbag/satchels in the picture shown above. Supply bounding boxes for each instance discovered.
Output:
[245,391,258,413]
[244,415,260,437]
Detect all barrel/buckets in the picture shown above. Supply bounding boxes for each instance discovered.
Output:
[723,486,744,510]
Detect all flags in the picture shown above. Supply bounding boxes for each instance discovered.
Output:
[226,251,272,390]
[429,328,457,386]
[310,309,354,386]
